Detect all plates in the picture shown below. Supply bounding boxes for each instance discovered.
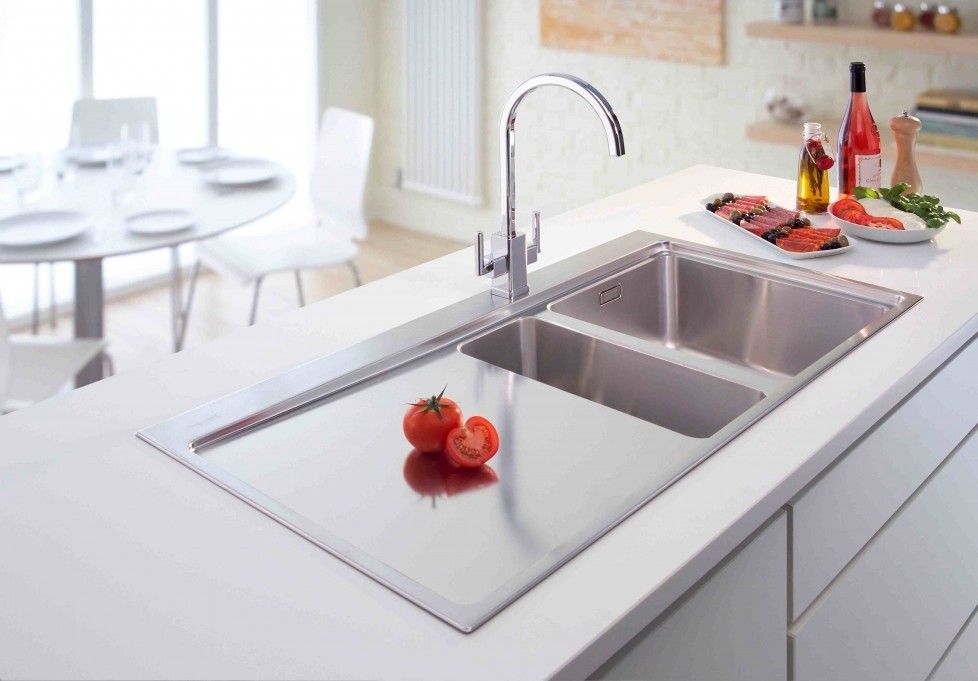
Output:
[204,158,281,186]
[0,209,91,246]
[0,156,28,171]
[66,146,125,163]
[828,199,949,243]
[697,192,859,260]
[178,147,236,163]
[126,209,196,234]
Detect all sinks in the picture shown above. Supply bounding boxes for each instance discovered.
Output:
[547,240,924,382]
[454,309,770,440]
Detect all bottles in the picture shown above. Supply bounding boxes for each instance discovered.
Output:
[837,61,882,199]
[890,110,923,197]
[871,1,914,31]
[919,3,960,33]
[796,122,836,215]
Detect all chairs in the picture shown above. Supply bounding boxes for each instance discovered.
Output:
[0,300,115,419]
[171,105,376,352]
[34,96,159,332]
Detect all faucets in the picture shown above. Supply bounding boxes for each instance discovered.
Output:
[474,68,625,298]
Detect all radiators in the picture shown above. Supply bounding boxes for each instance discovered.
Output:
[397,1,489,205]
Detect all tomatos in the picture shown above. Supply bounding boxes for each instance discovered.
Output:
[404,384,462,454]
[446,416,499,468]
[403,448,499,497]
[832,198,904,231]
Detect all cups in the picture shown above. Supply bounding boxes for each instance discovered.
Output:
[813,0,835,26]
[775,0,804,25]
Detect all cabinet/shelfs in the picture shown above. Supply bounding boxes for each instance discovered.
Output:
[586,333,978,680]
[745,17,978,170]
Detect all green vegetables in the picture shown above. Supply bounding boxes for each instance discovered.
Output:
[850,182,961,230]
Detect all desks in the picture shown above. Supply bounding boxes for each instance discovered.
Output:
[0,151,295,389]
